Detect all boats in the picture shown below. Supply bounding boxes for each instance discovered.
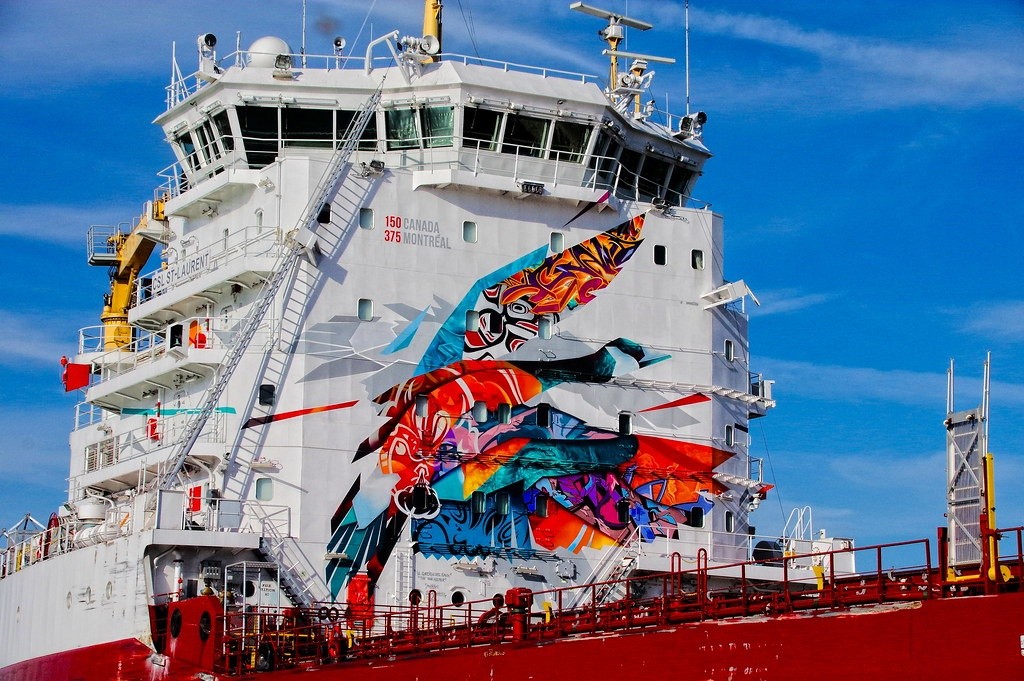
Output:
[0,0,1024,681]
